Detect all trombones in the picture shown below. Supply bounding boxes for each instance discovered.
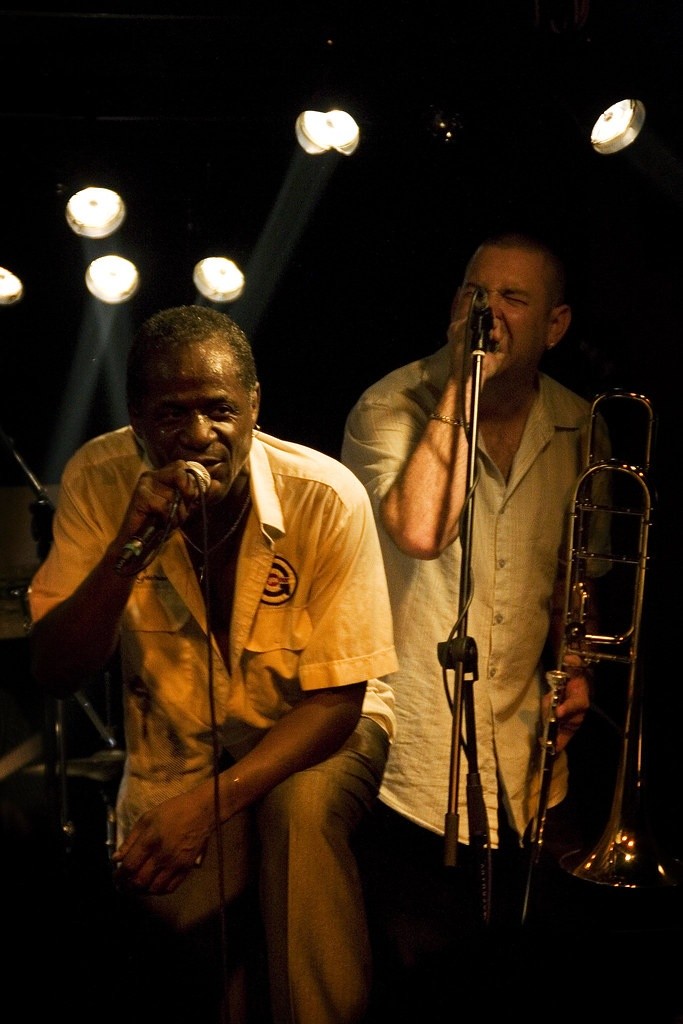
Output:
[520,385,682,926]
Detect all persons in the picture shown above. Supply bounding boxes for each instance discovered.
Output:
[340,234,615,1024]
[29,308,400,1024]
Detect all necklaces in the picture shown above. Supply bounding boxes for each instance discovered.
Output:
[178,493,251,589]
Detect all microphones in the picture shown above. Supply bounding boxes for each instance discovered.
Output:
[469,286,501,354]
[113,459,213,572]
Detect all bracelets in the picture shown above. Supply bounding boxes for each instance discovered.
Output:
[428,411,469,429]
